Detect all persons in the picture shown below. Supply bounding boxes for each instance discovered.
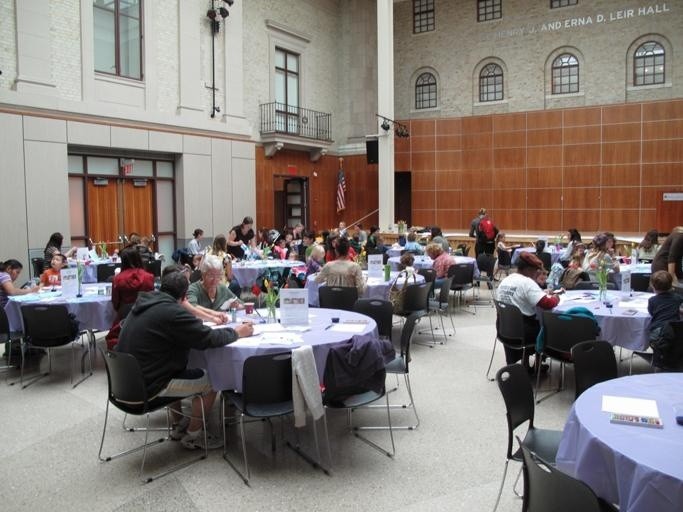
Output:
[0,259,42,361]
[492,250,559,380]
[192,254,202,270]
[187,255,247,321]
[162,264,224,325]
[113,269,255,451]
[471,207,500,290]
[106,249,154,355]
[494,220,683,295]
[646,271,682,349]
[33,216,451,295]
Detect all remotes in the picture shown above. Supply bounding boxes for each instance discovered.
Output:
[609,413,665,428]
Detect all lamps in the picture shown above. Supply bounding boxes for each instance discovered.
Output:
[375,113,410,138]
[205,1,233,37]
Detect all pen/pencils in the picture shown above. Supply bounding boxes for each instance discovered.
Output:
[242,321,256,325]
[325,324,333,330]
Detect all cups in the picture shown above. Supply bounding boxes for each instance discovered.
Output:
[243,302,255,315]
[35,278,40,292]
[384,265,390,281]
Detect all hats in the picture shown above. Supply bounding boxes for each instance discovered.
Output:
[521,251,543,269]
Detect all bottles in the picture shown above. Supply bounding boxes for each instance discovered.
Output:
[240,244,248,252]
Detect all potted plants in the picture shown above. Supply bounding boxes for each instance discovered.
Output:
[397,220,406,233]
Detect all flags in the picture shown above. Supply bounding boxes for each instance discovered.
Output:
[336,167,347,213]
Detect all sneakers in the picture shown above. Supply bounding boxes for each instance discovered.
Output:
[170,416,190,440]
[181,429,224,450]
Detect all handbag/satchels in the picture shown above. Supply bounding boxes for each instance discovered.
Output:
[389,270,416,316]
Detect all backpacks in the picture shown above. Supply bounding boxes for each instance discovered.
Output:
[478,215,495,241]
